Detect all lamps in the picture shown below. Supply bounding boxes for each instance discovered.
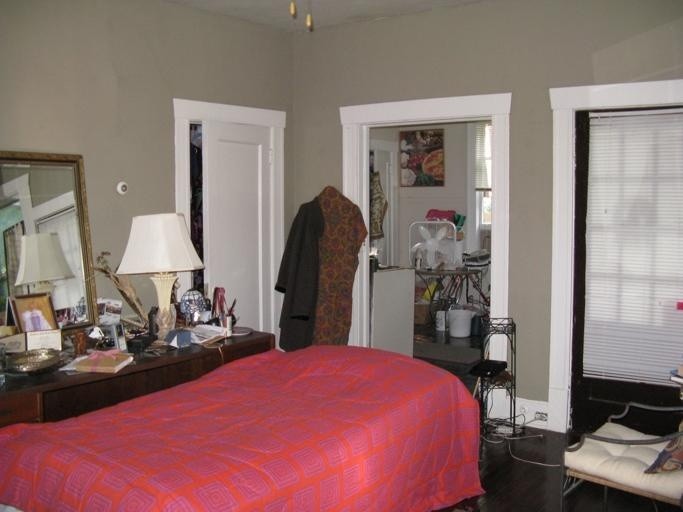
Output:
[289,0,313,31]
[14,232,74,292]
[116,212,204,331]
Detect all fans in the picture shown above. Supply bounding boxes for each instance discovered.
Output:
[408,218,457,269]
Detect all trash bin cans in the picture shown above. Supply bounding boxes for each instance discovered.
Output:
[448,304,476,338]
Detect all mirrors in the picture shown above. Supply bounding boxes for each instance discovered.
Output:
[0,150,99,338]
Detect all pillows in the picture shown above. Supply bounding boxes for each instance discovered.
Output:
[644,418,683,472]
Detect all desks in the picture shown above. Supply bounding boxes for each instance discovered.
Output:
[408,270,489,339]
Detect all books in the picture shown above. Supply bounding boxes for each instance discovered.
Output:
[59,352,133,374]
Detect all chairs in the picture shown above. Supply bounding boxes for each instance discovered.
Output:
[561,403,683,512]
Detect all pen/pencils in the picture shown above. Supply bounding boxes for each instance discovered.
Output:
[229,298,236,316]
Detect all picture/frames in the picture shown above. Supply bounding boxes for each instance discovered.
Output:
[4,297,14,326]
[9,292,57,334]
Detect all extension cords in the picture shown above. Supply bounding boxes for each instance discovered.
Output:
[495,425,523,434]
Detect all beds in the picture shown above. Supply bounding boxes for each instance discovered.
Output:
[1,345,484,512]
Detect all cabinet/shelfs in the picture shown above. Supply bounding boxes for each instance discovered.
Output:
[1,328,275,426]
[370,268,416,357]
